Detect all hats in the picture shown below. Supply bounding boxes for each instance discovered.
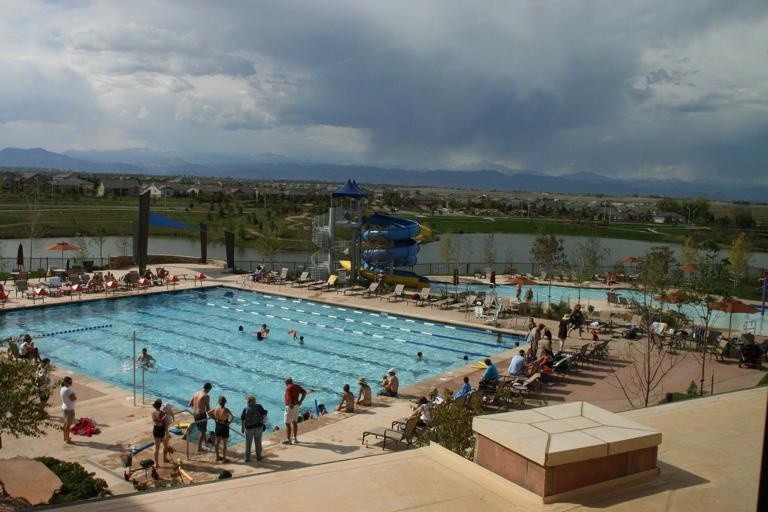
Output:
[562,314,570,320]
[388,368,396,373]
[357,378,368,384]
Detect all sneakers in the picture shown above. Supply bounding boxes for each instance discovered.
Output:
[246,459,250,463]
[281,440,292,445]
[221,459,230,464]
[292,437,297,444]
[257,456,263,461]
[216,457,224,461]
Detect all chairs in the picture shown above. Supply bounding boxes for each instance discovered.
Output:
[0,268,179,305]
[496,339,613,396]
[362,388,487,451]
[247,264,289,287]
[580,316,767,371]
[291,272,529,326]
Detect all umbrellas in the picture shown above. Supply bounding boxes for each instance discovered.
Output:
[706,295,760,342]
[680,263,704,284]
[617,257,642,275]
[505,275,535,299]
[652,287,699,332]
[16,243,24,271]
[43,240,81,268]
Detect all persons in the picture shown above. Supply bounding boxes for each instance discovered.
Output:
[508,284,585,377]
[455,376,471,400]
[64,265,171,292]
[252,266,263,282]
[137,348,156,369]
[476,358,498,391]
[151,368,399,468]
[417,352,424,363]
[259,324,267,337]
[404,396,435,428]
[59,376,77,444]
[37,358,51,416]
[293,335,304,344]
[19,334,42,362]
[256,331,267,340]
[495,333,505,343]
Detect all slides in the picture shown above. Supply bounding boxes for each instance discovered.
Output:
[339,211,432,289]
